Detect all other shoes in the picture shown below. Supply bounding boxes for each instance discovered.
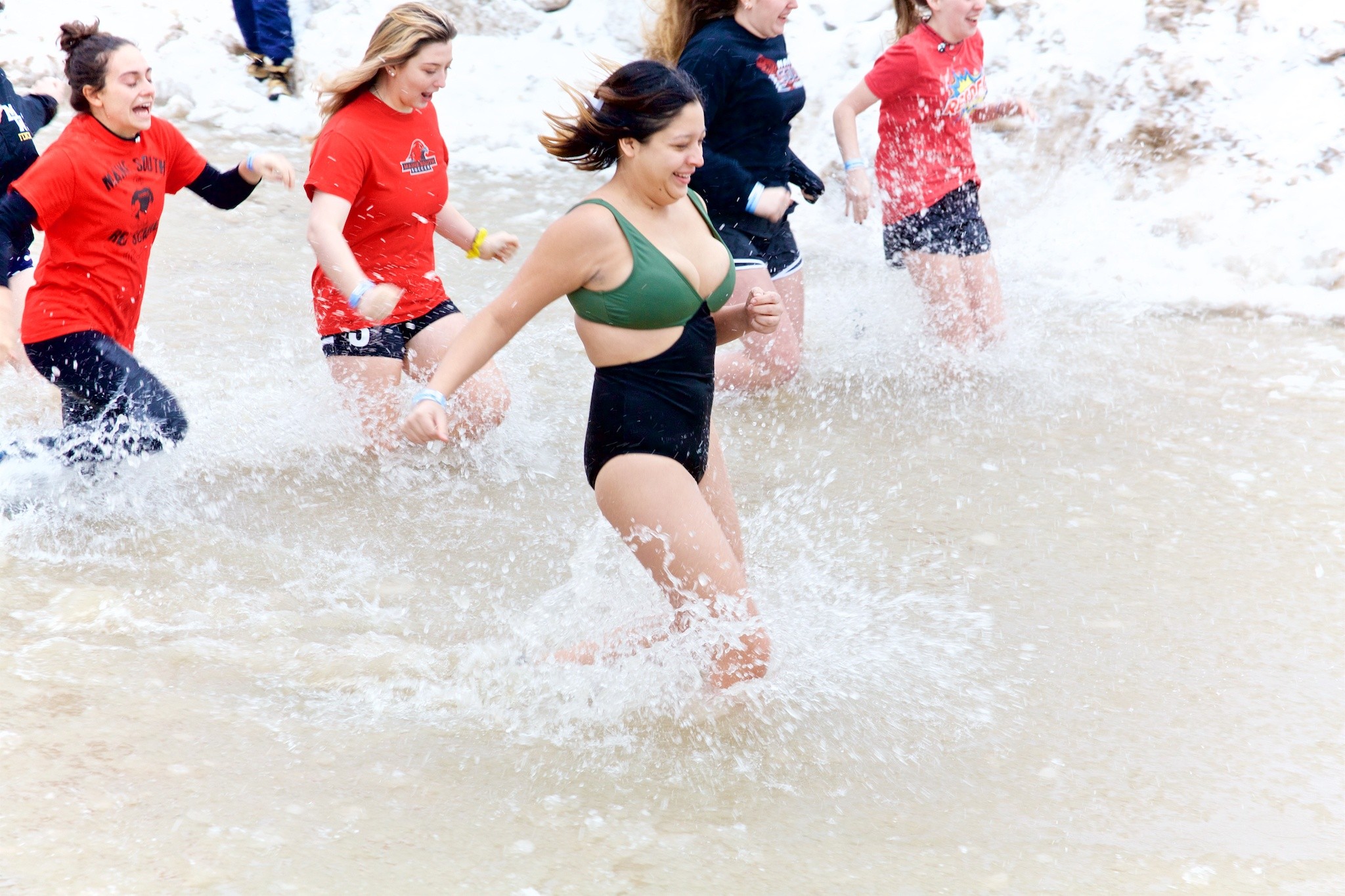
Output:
[263,56,293,97]
[247,52,268,79]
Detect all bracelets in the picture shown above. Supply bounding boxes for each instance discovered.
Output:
[746,181,765,214]
[844,158,864,170]
[467,227,488,259]
[247,154,256,170]
[347,280,375,308]
[412,389,447,408]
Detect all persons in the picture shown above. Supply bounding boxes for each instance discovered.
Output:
[303,0,520,456]
[643,1,824,393]
[232,0,295,102]
[397,61,782,692]
[0,3,64,372]
[1,13,297,469]
[833,0,1037,354]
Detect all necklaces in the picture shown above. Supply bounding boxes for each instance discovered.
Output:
[920,13,965,58]
